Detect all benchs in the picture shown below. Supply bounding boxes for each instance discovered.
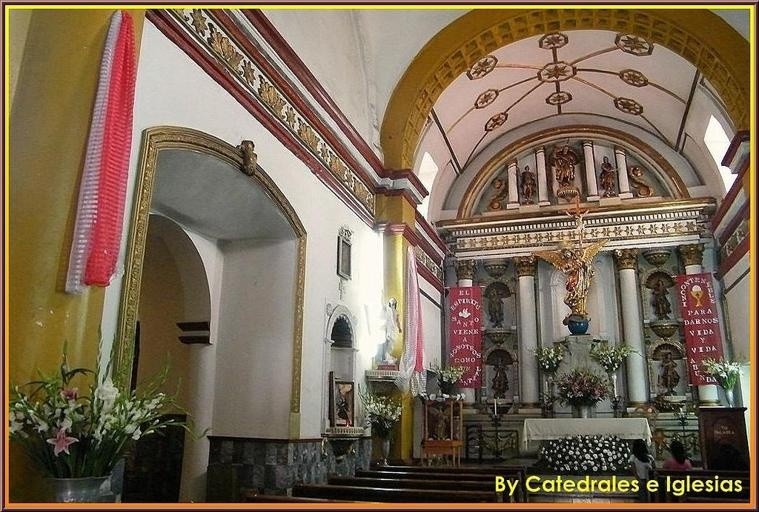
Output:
[240,463,530,504]
[648,468,750,504]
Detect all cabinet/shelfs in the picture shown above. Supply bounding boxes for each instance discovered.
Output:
[695,405,749,471]
[419,394,466,468]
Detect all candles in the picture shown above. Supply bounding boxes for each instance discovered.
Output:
[680,405,684,418]
[493,398,497,416]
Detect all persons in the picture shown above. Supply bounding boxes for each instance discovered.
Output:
[628,440,658,503]
[479,142,687,403]
[663,441,694,473]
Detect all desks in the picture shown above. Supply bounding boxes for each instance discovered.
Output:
[519,417,653,448]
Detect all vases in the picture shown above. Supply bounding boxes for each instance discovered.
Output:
[724,389,735,406]
[608,373,618,397]
[577,404,590,418]
[447,382,454,395]
[546,373,553,397]
[43,474,119,502]
[380,439,390,466]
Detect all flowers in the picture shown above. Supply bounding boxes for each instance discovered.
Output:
[529,344,567,375]
[11,327,216,478]
[701,358,740,390]
[359,387,411,438]
[438,365,467,384]
[590,342,646,375]
[555,366,610,408]
